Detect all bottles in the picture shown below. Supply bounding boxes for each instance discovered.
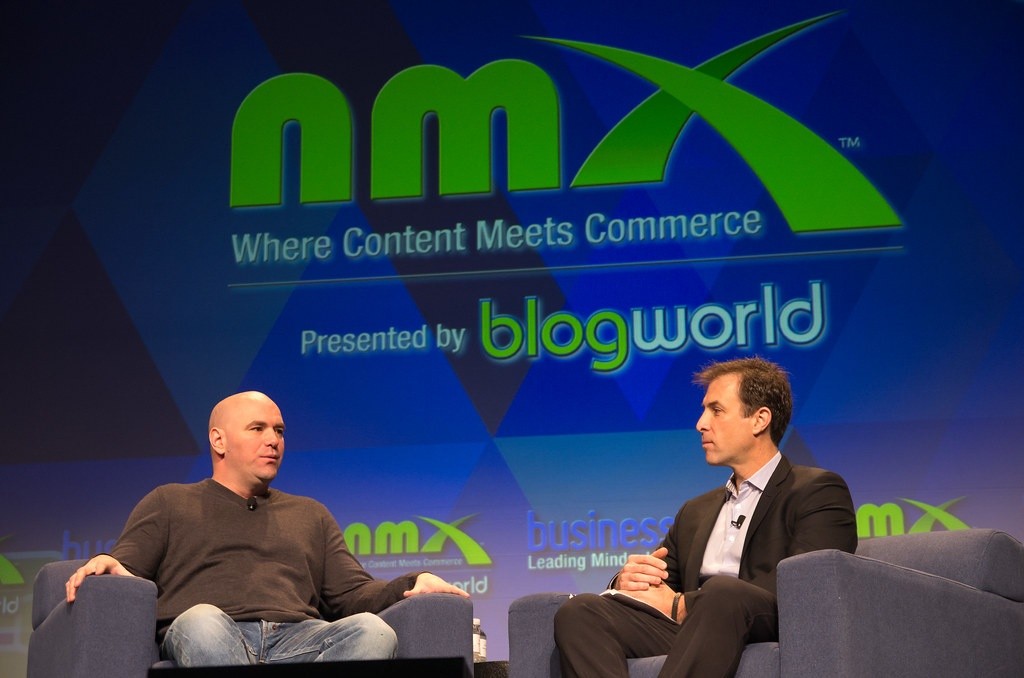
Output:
[473,618,487,663]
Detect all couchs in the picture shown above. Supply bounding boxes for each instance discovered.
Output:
[507,529,1024,678]
[25,558,474,678]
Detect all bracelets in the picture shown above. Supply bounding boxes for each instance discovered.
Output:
[670,592,682,621]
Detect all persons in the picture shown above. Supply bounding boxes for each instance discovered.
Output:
[551,358,860,677]
[65,391,470,668]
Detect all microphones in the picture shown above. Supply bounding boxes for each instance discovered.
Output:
[247,497,257,510]
[735,514,746,529]
